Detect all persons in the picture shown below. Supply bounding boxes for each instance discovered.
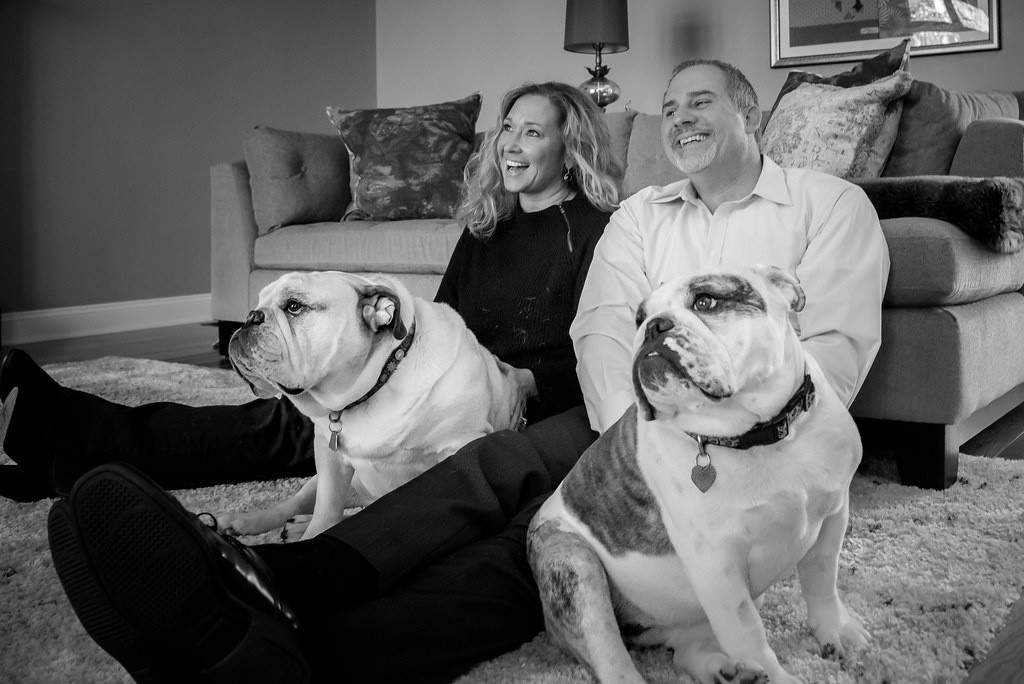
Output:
[0,80,620,501]
[50,61,889,684]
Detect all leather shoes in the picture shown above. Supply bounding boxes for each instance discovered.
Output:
[47,498,158,684]
[69,460,312,683]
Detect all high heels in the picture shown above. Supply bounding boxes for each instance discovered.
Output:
[1,346,62,427]
[0,384,60,504]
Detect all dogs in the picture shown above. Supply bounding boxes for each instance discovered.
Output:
[209,267,514,542]
[526,267,876,684]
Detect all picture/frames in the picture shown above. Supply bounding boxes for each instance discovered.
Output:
[769,0,1001,68]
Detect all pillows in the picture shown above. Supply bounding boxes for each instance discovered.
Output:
[326,90,483,221]
[760,38,913,179]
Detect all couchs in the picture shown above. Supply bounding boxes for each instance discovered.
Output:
[201,81,1023,489]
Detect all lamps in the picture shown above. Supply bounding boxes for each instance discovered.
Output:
[564,0,629,112]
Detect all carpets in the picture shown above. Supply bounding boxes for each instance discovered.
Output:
[0,355,1024,684]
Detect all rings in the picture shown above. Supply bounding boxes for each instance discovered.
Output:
[520,417,527,428]
